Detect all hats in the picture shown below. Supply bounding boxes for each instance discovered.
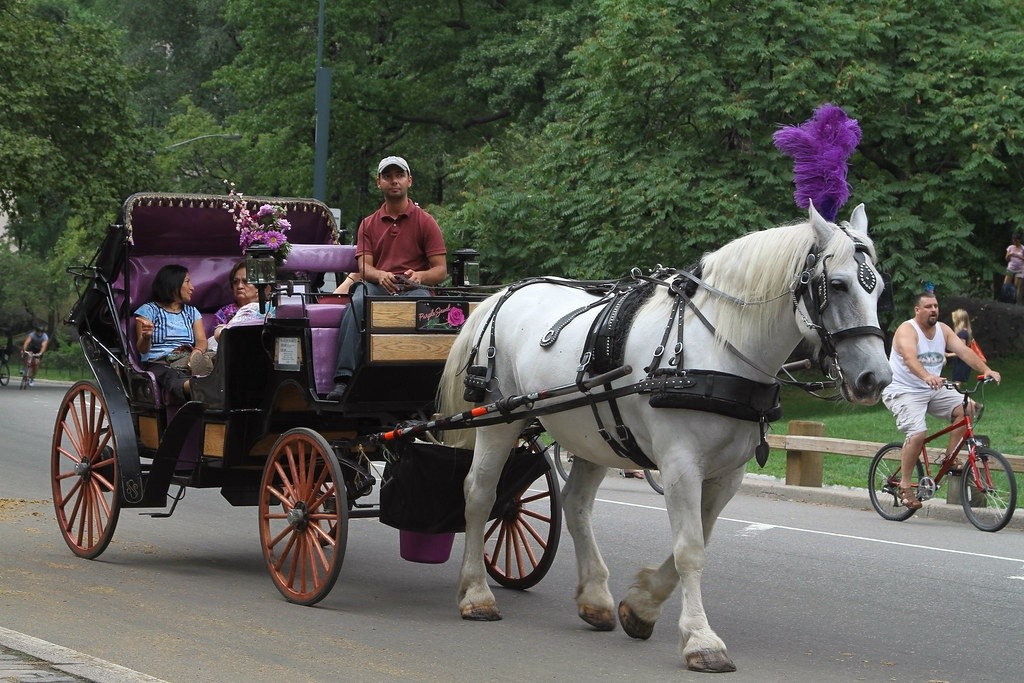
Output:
[378,156,410,178]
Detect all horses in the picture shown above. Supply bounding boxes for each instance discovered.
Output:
[431,198,891,673]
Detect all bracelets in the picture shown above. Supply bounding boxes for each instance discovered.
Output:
[194,347,204,353]
[214,324,225,329]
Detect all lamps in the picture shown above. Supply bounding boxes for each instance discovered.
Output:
[452,242,480,285]
[242,239,276,284]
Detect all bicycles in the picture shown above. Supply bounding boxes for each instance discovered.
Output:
[19,352,35,389]
[0,352,10,386]
[867,373,1018,534]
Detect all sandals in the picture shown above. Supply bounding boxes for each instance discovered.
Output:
[896,485,922,510]
[942,457,966,473]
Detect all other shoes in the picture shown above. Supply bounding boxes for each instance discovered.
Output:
[29,378,34,386]
[20,370,24,374]
[190,351,214,378]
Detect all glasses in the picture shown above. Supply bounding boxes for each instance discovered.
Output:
[231,279,247,285]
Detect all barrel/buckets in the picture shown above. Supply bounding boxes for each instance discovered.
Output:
[400,530,455,563]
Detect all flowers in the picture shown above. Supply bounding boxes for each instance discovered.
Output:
[220,178,292,266]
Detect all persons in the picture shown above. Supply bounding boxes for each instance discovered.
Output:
[1004,231,1024,304]
[328,156,447,406]
[881,291,1002,510]
[21,326,48,387]
[209,261,275,366]
[135,264,208,429]
[945,308,972,393]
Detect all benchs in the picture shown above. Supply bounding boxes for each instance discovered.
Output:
[265,243,359,326]
[114,252,245,410]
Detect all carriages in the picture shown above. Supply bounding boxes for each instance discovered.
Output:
[50,191,893,672]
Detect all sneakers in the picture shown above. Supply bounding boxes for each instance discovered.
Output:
[327,381,347,401]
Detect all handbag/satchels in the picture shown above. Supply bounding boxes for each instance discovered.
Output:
[968,336,987,364]
[999,284,1017,304]
